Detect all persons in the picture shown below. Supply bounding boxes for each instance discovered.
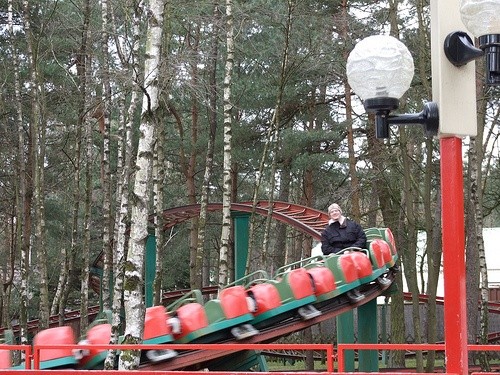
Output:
[320,203,367,258]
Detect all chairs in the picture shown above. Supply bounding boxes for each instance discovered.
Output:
[0,226,398,373]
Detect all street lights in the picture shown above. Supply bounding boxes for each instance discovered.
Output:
[345,1,500,375]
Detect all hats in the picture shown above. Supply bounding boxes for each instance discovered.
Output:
[328,203,342,217]
